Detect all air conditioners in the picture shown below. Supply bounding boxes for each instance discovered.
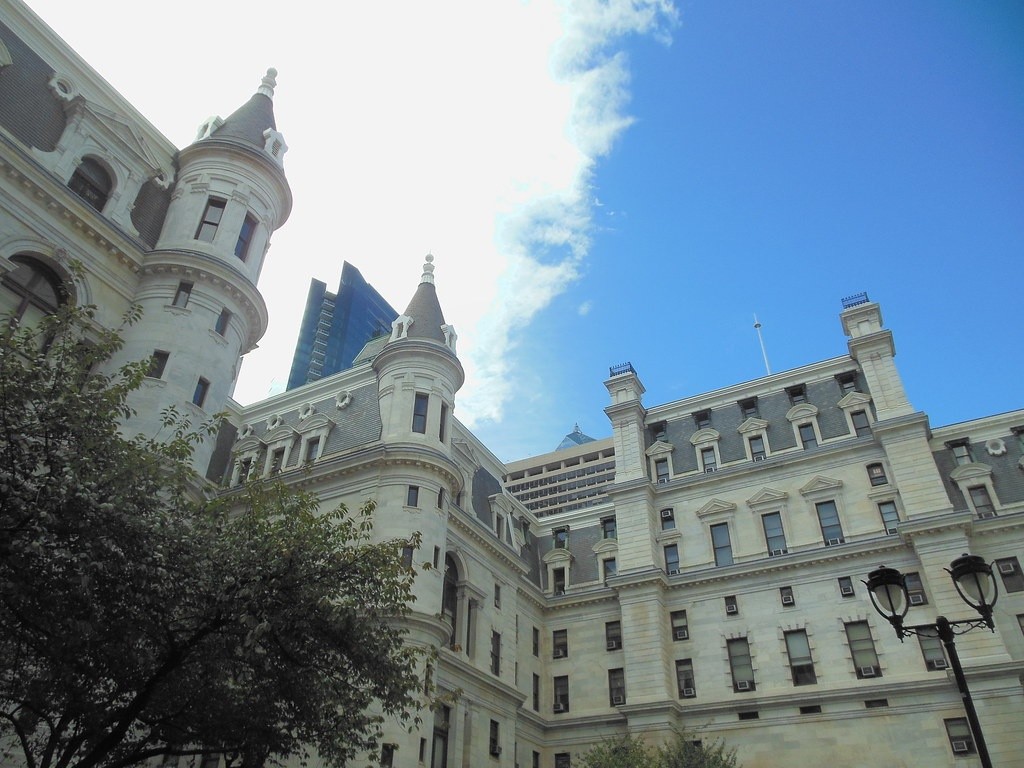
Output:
[909,594,923,605]
[782,596,794,604]
[888,527,899,535]
[491,745,502,754]
[657,478,667,484]
[553,649,564,657]
[684,688,695,696]
[828,538,840,546]
[553,703,563,711]
[952,740,967,752]
[705,467,714,473]
[670,569,679,575]
[999,564,1014,574]
[753,456,764,462]
[661,510,672,518]
[676,630,688,639]
[841,587,853,594]
[773,549,782,556]
[607,641,617,649]
[738,681,750,689]
[555,590,564,596]
[613,695,624,704]
[982,512,995,518]
[934,659,948,668]
[861,666,875,677]
[726,604,736,613]
[495,599,501,608]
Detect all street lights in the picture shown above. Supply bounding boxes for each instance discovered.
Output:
[859,552,998,768]
